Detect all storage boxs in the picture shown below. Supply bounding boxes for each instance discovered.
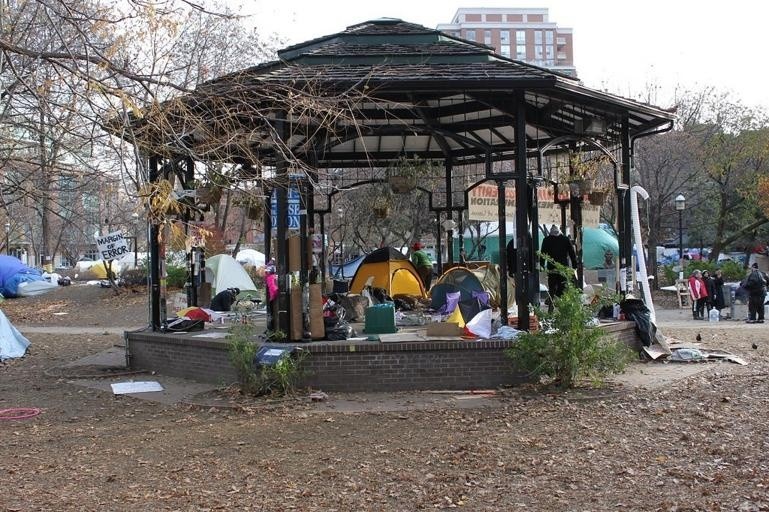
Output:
[364,307,398,334]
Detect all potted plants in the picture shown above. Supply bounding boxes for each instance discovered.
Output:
[388,157,444,194]
[232,191,262,219]
[188,170,229,204]
[371,197,389,218]
[589,184,610,206]
[568,153,606,196]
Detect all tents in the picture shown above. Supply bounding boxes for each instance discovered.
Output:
[349,245,426,308]
[428,259,518,316]
[230,246,265,270]
[74,246,188,279]
[409,242,434,292]
[0,253,55,297]
[331,253,370,276]
[183,252,262,303]
[0,307,33,363]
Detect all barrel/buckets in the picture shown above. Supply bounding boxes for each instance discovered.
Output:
[709,306,720,322]
[364,303,397,334]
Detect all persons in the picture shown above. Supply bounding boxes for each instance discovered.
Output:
[686,269,707,320]
[741,261,769,323]
[507,230,542,318]
[710,269,725,309]
[699,270,718,319]
[539,223,579,316]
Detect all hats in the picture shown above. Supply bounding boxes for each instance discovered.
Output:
[751,263,759,269]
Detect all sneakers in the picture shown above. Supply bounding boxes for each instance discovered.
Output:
[693,317,704,319]
[745,319,765,323]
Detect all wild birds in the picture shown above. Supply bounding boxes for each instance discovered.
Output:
[696,333,701,341]
[752,343,758,350]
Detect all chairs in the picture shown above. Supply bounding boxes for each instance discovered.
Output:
[322,279,349,320]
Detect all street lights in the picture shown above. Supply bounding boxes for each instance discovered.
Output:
[674,194,686,280]
[337,206,343,279]
